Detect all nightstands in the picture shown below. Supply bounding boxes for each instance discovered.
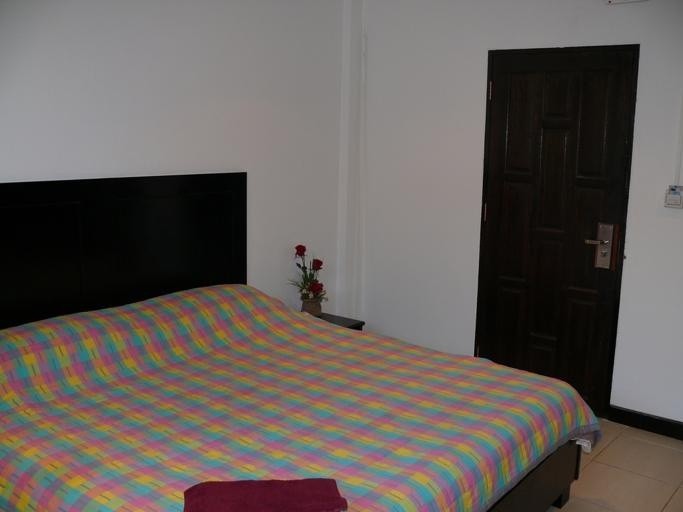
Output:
[318,312,366,332]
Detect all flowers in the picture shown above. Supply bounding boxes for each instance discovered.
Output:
[285,243,329,302]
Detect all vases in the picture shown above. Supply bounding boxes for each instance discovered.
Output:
[301,297,324,317]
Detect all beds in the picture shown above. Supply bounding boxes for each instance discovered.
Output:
[0,173,602,512]
